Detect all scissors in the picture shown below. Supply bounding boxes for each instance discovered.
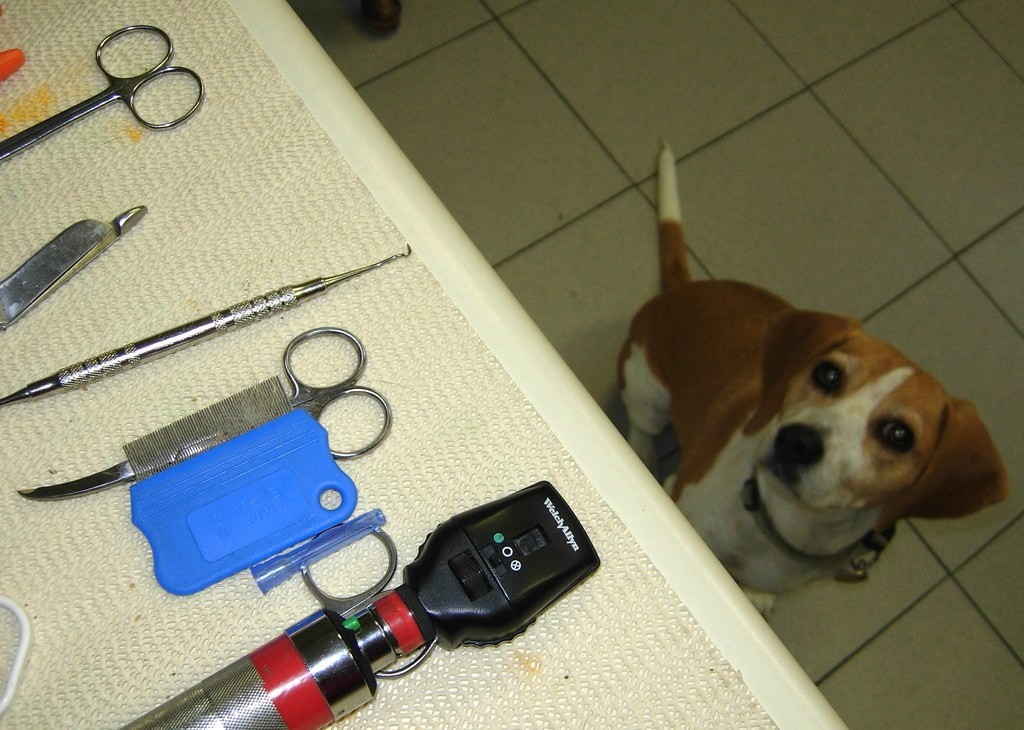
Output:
[15,324,398,502]
[300,523,439,678]
[1,26,205,163]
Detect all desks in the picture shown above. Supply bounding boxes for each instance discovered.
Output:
[0,0,848,730]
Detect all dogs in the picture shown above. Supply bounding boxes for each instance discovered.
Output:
[613,132,1011,622]
